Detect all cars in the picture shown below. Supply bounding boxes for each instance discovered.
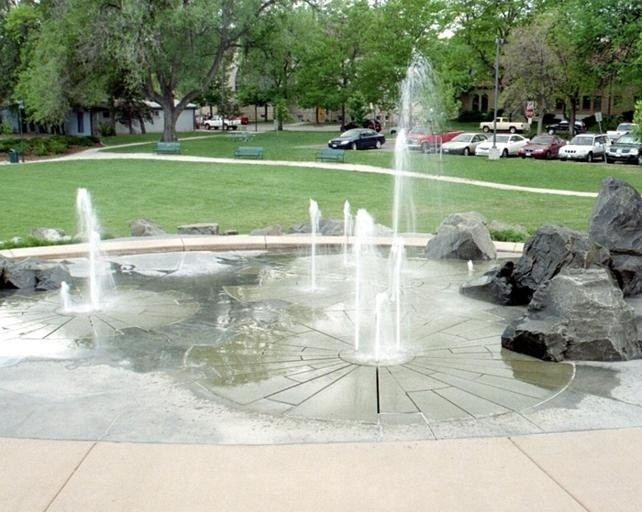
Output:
[194,109,251,132]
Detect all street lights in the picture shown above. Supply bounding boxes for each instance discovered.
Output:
[16,98,26,162]
[488,35,506,161]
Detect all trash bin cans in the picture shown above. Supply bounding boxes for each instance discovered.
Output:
[298,116,304,122]
[8,152,19,163]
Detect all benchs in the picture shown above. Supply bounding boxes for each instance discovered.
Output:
[316,149,344,162]
[153,143,181,154]
[233,146,264,159]
